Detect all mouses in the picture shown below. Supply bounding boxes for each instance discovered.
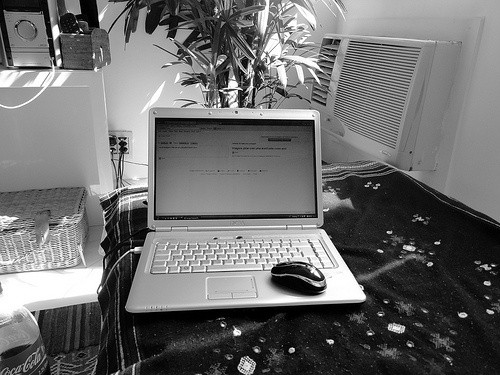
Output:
[271,261,327,294]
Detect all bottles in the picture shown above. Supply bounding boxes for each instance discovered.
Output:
[0,283,51,375]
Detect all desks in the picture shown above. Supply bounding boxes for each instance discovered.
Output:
[109,156,500,374]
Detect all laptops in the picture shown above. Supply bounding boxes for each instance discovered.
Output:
[123,107,366,313]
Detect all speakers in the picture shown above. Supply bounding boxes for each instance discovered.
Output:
[0,0,63,67]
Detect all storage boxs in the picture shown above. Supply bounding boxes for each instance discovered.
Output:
[0,186,88,274]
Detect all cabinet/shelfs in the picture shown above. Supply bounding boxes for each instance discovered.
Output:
[0,70,115,312]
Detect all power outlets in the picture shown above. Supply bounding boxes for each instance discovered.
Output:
[107,132,133,157]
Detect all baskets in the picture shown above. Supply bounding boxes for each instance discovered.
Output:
[0,187,89,275]
